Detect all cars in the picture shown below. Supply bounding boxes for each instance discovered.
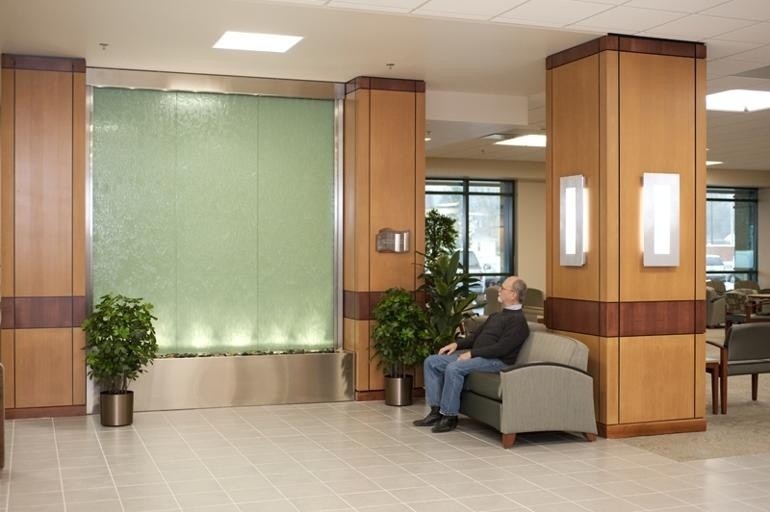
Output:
[706,254,727,282]
[456,249,491,294]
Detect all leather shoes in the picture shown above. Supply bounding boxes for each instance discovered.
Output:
[414,413,442,426]
[432,415,458,432]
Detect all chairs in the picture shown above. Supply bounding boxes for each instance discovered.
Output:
[706,279,770,414]
[483,285,545,323]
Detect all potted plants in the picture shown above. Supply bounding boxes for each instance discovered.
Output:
[78,291,160,427]
[370,286,437,406]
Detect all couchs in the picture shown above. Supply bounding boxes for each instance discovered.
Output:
[460,331,600,449]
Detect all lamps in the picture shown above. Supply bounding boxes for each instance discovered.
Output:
[375,227,411,254]
[558,171,682,268]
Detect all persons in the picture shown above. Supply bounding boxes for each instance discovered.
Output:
[412,276,530,433]
